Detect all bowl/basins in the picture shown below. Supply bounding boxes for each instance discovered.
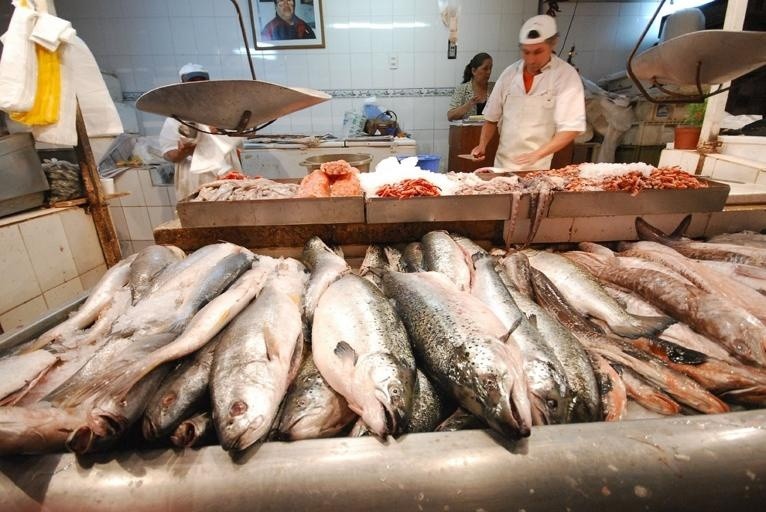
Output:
[299,151,374,175]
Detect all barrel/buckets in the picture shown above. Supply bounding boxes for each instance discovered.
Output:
[300,152,373,179]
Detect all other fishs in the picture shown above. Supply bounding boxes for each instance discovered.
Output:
[0,212,761,457]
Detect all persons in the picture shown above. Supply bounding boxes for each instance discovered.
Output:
[159,64,243,203]
[446,52,496,121]
[261,0,316,42]
[469,15,586,172]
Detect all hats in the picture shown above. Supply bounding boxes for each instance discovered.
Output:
[518,14,557,44]
[178,62,208,82]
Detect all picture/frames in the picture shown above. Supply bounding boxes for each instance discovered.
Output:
[248,0,325,50]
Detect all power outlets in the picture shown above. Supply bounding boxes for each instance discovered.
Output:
[388,55,399,69]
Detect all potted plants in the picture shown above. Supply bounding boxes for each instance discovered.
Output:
[674,99,707,150]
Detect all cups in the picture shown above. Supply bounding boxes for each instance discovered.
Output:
[100,176,116,196]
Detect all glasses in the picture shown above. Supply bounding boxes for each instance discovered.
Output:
[276,0,293,6]
